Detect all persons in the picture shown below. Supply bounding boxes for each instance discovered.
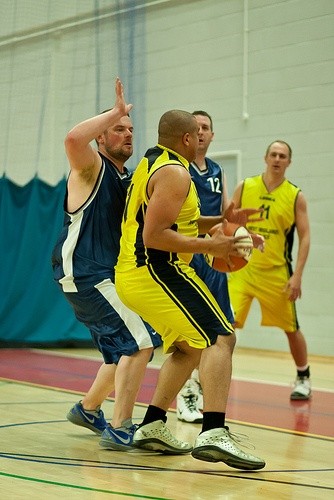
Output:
[177,110,264,423]
[51,77,162,451]
[225,140,311,400]
[114,110,266,470]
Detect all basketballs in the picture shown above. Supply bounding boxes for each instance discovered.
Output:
[202,222,253,272]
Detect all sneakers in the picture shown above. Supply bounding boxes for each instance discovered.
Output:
[176,388,203,422]
[290,380,311,399]
[132,416,193,453]
[100,423,139,450]
[190,377,204,410]
[66,400,110,437]
[191,426,265,470]
